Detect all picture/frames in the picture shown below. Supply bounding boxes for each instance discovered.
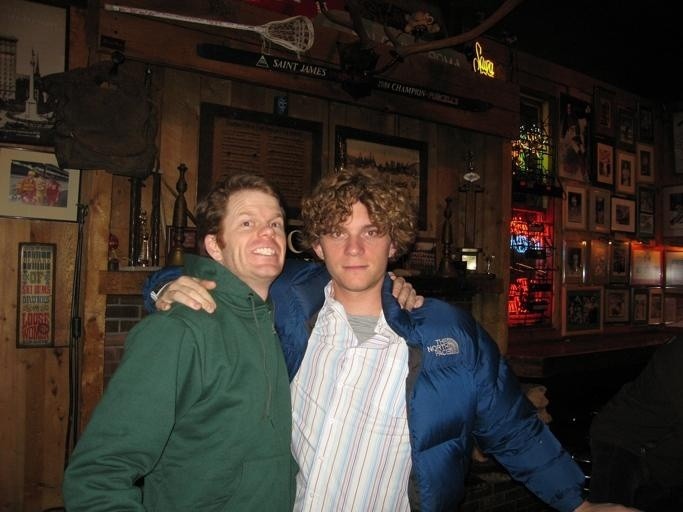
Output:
[332,122,431,230]
[0,144,83,223]
[556,82,682,337]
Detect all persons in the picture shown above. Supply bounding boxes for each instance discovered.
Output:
[562,102,652,327]
[18,169,60,207]
[590,338,683,511]
[144,162,642,512]
[61,172,427,512]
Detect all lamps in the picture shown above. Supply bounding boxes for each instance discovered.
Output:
[330,10,397,102]
